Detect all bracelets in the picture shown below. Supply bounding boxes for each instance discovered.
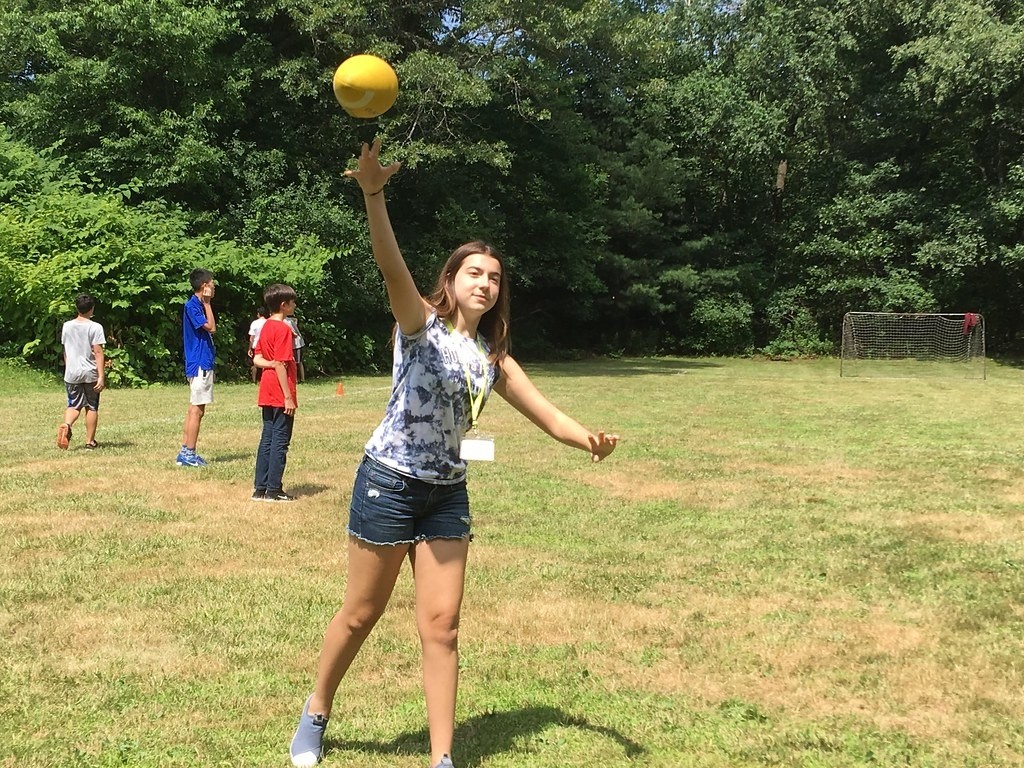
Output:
[366,189,382,196]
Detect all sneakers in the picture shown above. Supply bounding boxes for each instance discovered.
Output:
[85,439,104,449]
[57,423,73,450]
[291,693,332,767]
[265,489,297,501]
[430,754,455,768]
[182,456,208,467]
[177,452,184,466]
[251,488,266,501]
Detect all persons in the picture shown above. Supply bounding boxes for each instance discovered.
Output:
[175,269,216,466]
[283,316,305,384]
[247,306,267,383]
[252,284,298,503]
[56,296,106,451]
[289,139,621,768]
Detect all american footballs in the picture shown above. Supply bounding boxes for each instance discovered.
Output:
[333,54,400,118]
